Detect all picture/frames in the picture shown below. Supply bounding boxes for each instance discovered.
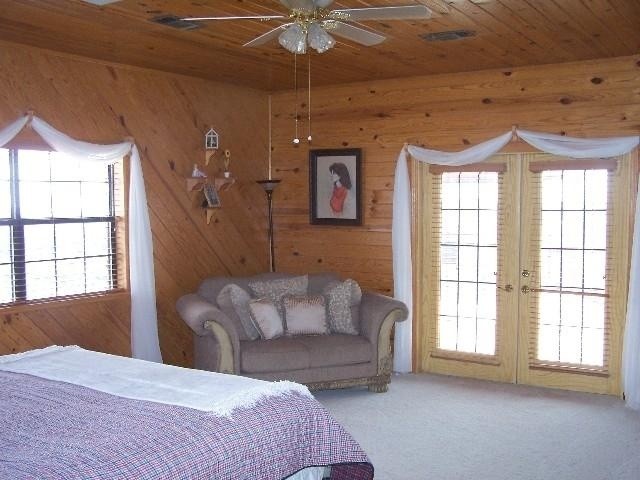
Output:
[204,185,221,207]
[308,147,363,226]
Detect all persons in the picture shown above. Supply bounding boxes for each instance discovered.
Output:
[328,163,352,218]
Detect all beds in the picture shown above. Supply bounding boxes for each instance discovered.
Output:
[1,342,330,480]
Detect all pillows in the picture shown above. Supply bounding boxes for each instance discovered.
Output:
[216,272,363,342]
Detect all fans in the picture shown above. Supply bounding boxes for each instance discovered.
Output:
[181,0,431,48]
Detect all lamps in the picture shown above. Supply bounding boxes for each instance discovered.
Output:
[278,20,336,55]
[256,179,281,271]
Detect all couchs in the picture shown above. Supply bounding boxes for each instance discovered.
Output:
[176,272,408,394]
